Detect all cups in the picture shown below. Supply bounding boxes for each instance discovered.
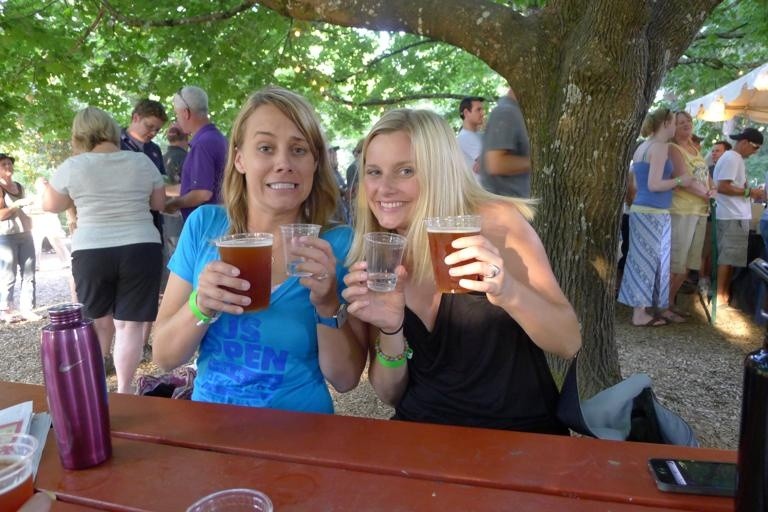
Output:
[361,230,408,293]
[212,224,324,312]
[422,214,480,293]
[0,431,39,511]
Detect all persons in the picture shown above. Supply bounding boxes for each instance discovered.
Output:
[326,136,366,224]
[153,88,368,414]
[44,87,233,396]
[616,106,768,327]
[0,153,46,326]
[455,87,531,196]
[341,109,584,436]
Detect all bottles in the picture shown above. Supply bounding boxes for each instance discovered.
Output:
[39,302,112,470]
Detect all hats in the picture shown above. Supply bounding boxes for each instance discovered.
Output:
[690,133,703,143]
[729,128,763,145]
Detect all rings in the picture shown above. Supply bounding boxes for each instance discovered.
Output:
[480,263,501,280]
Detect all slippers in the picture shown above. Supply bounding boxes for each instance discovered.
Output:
[631,307,691,327]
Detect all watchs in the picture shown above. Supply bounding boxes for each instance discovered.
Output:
[312,304,349,329]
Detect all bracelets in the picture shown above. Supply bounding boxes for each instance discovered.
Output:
[373,338,414,367]
[373,320,406,335]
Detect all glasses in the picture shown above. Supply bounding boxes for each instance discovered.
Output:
[177,86,191,109]
[748,141,760,149]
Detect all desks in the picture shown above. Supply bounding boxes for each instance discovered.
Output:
[1,379,741,512]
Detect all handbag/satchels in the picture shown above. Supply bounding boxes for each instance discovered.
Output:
[562,355,701,448]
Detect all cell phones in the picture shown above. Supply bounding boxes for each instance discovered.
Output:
[648,458,738,497]
